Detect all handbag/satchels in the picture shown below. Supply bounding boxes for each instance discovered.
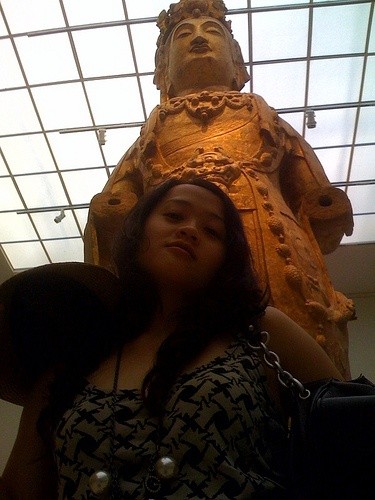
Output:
[243,321,374,500]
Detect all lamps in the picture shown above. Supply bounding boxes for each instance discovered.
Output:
[53,209,65,223]
[97,129,106,145]
[305,109,317,129]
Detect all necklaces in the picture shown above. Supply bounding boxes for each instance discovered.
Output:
[89,336,178,500]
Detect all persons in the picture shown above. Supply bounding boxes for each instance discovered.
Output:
[0,180,375,500]
[84,1,357,383]
